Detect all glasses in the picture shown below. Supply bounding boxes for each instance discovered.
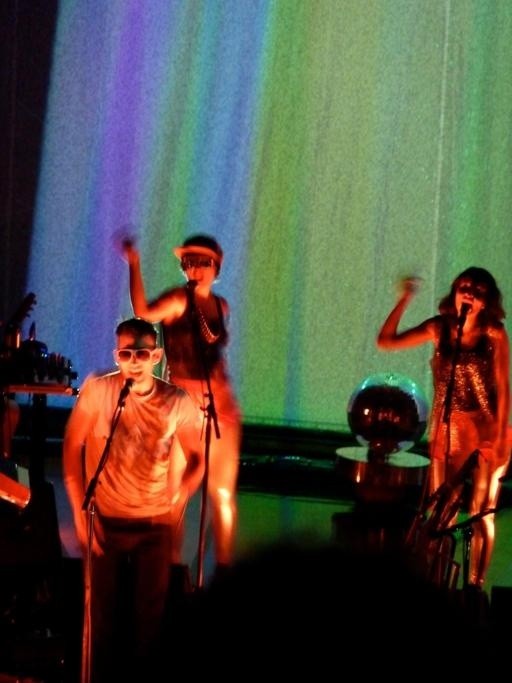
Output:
[180,258,212,271]
[112,345,160,365]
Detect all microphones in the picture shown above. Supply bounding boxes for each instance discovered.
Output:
[118,377,135,406]
[456,303,470,327]
[185,280,198,291]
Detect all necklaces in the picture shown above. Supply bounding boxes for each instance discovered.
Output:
[194,295,220,343]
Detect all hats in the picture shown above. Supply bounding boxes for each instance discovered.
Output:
[173,236,223,263]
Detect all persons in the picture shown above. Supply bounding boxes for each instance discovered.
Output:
[377,264,509,589]
[63,317,210,682]
[116,226,242,570]
